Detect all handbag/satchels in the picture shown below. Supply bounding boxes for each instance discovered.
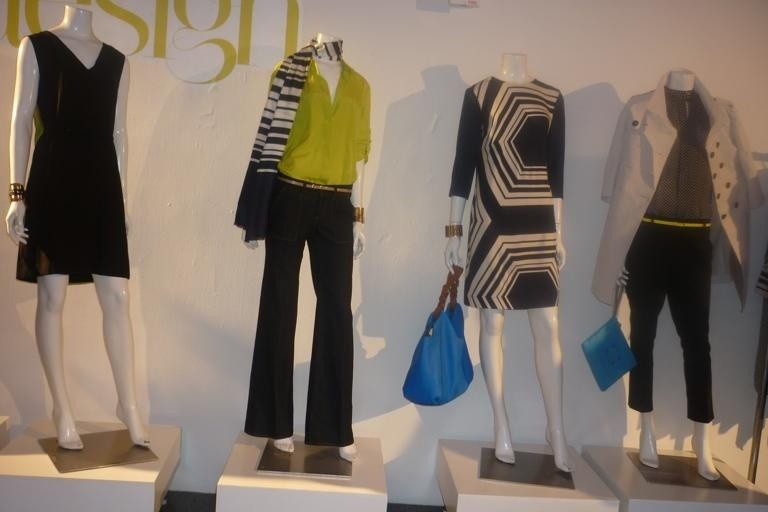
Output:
[402,303,473,406]
[582,317,637,392]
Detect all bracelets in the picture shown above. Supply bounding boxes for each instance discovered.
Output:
[350,206,364,223]
[447,226,463,239]
[10,183,24,202]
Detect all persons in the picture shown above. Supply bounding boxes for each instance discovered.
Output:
[614,69,725,482]
[238,31,372,461]
[445,51,573,472]
[4,3,150,448]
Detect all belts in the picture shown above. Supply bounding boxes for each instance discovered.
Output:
[641,217,711,227]
[277,176,352,193]
